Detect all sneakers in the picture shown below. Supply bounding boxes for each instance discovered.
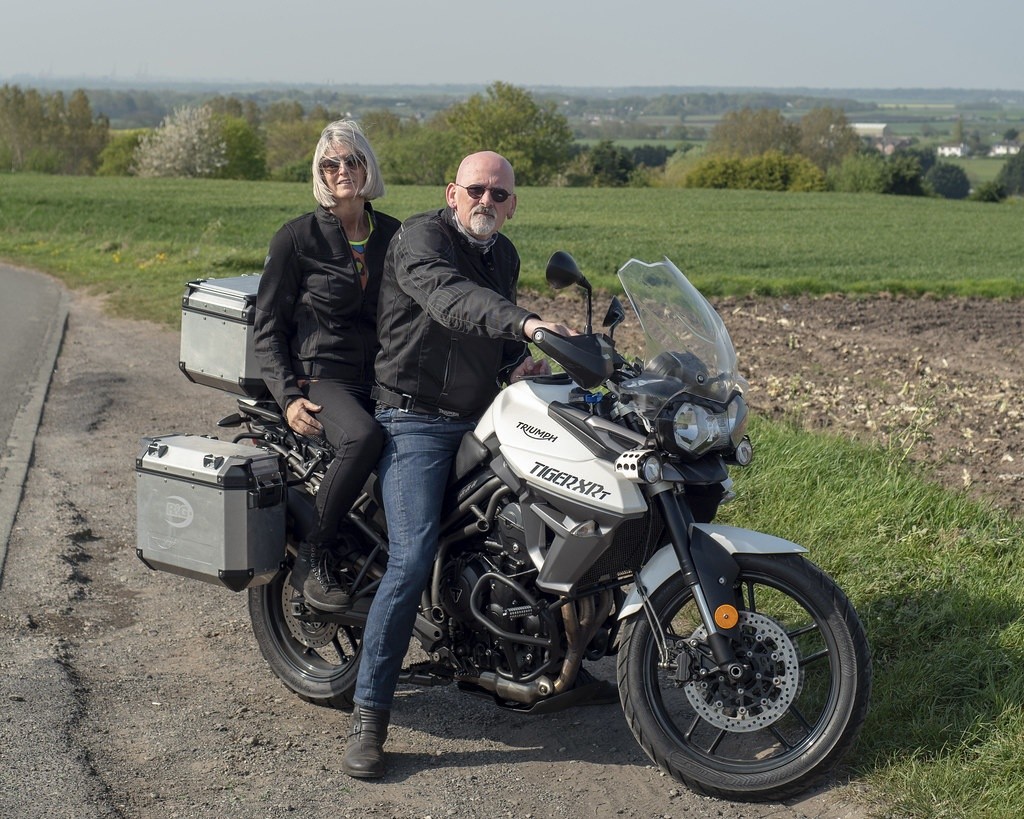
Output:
[344,704,389,780]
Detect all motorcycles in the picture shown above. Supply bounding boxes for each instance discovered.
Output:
[134,249,875,806]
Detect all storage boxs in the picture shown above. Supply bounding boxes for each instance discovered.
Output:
[178,271,268,400]
[134,431,287,590]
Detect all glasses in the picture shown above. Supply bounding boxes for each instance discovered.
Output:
[321,154,365,174]
[455,184,513,202]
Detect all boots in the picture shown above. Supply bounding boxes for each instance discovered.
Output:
[287,540,351,611]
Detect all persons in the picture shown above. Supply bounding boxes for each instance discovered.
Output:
[342,150,572,778]
[252,116,403,613]
